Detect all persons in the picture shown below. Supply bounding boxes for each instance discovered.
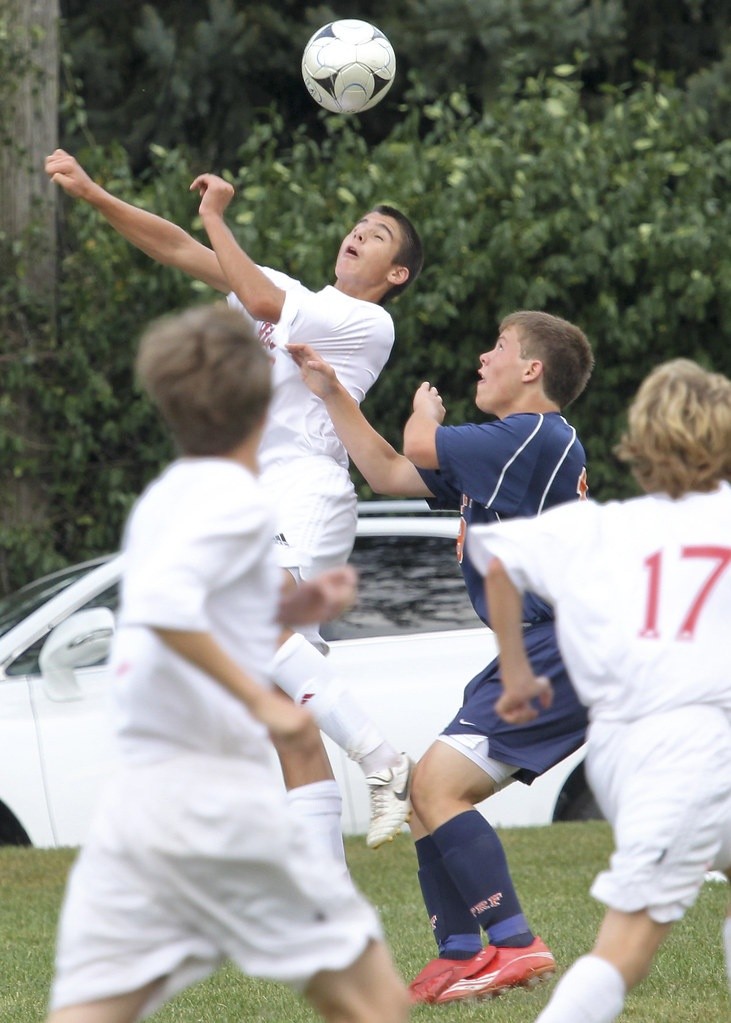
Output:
[44,302,418,1023]
[284,311,596,1003]
[43,149,416,864]
[466,354,731,1021]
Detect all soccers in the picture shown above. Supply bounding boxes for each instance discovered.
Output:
[302,19,396,115]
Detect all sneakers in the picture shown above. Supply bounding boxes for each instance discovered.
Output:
[365,753,422,850]
[408,950,484,1006]
[432,936,557,1004]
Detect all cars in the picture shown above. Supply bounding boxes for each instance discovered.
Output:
[0,498,612,850]
